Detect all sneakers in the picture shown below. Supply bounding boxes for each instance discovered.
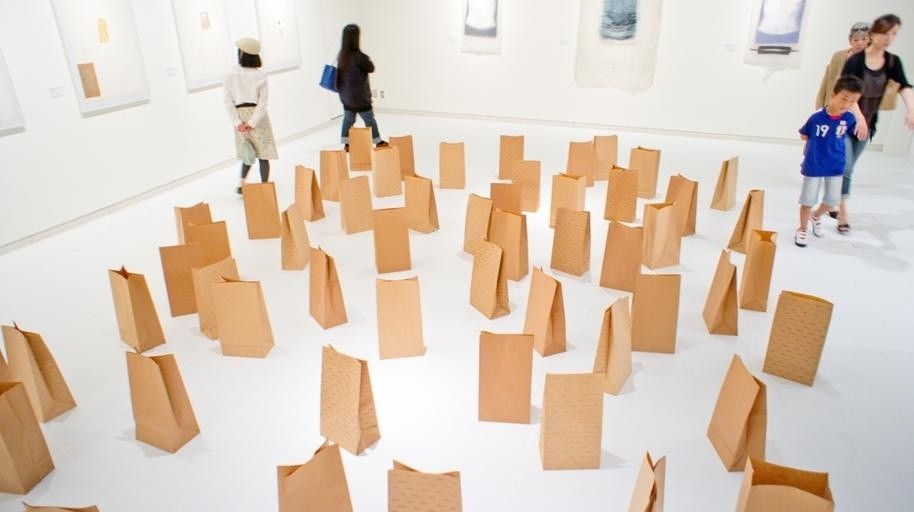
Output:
[810,217,824,236]
[796,229,808,246]
[344,147,349,152]
[376,141,388,147]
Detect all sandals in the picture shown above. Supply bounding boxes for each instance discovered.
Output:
[838,224,850,233]
[828,210,840,219]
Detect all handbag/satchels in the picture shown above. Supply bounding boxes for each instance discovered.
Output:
[878,78,901,111]
[319,64,338,92]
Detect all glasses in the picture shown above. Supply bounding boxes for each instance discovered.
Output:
[852,27,869,32]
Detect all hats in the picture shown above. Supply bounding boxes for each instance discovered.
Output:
[850,22,870,36]
[234,37,260,55]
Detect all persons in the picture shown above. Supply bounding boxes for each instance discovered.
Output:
[815,21,872,112]
[336,23,389,152]
[825,14,914,234]
[794,74,866,248]
[221,37,279,194]
